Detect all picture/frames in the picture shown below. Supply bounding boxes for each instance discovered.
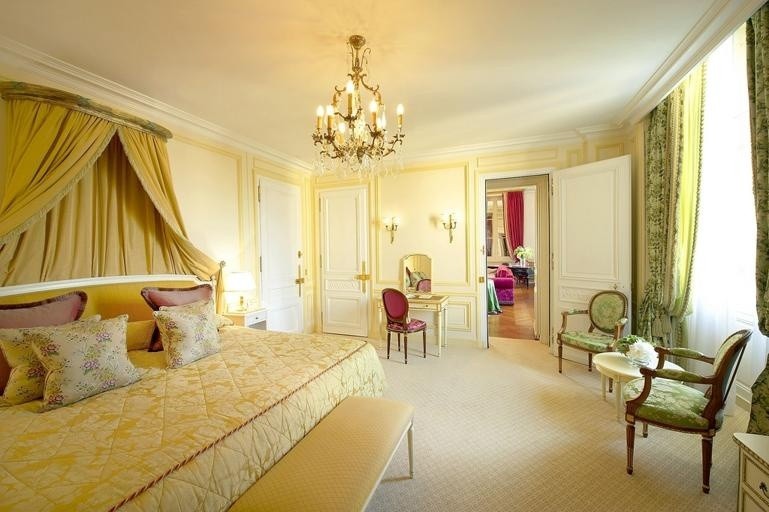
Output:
[376,292,447,357]
[488,265,528,283]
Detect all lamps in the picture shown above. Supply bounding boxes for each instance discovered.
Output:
[556,290,628,393]
[621,329,751,493]
[417,279,431,293]
[381,288,426,364]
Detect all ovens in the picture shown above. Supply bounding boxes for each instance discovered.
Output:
[0,81,387,511]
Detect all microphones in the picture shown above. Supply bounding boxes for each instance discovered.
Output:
[518,272,529,288]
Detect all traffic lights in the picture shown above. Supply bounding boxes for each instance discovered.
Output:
[14,315,142,414]
[216,313,233,330]
[411,271,427,288]
[126,320,157,352]
[0,291,87,398]
[151,306,221,369]
[0,314,102,407]
[140,284,213,352]
[159,300,221,344]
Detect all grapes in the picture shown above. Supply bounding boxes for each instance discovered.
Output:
[223,308,269,331]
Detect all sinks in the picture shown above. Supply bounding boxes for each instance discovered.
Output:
[487,265,514,306]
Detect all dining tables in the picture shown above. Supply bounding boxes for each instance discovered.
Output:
[615,335,640,356]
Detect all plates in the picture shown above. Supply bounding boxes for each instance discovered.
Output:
[732,432,768,512]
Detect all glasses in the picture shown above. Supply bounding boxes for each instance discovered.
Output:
[225,271,256,312]
[440,207,456,242]
[383,215,401,243]
[310,34,405,161]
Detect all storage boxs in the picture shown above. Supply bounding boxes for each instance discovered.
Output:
[226,398,414,510]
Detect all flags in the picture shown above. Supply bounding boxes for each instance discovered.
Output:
[520,258,525,267]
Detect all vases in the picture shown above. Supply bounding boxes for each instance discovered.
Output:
[629,339,657,362]
[513,246,532,259]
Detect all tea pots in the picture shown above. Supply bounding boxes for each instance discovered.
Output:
[400,253,431,293]
